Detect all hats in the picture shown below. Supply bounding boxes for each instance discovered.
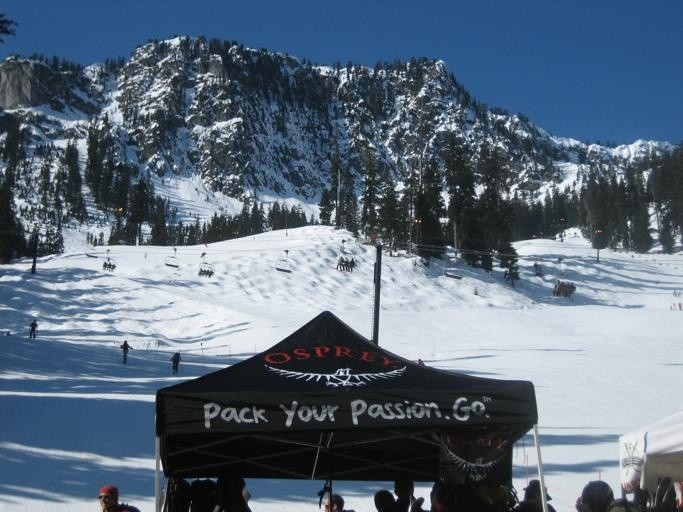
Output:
[100,486,118,498]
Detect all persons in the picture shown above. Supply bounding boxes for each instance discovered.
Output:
[323,495,355,512]
[393,479,428,512]
[335,256,344,271]
[346,257,358,272]
[342,257,349,271]
[373,490,403,512]
[119,340,133,364]
[574,480,637,512]
[169,352,181,375]
[97,484,140,512]
[512,480,556,512]
[27,319,38,339]
